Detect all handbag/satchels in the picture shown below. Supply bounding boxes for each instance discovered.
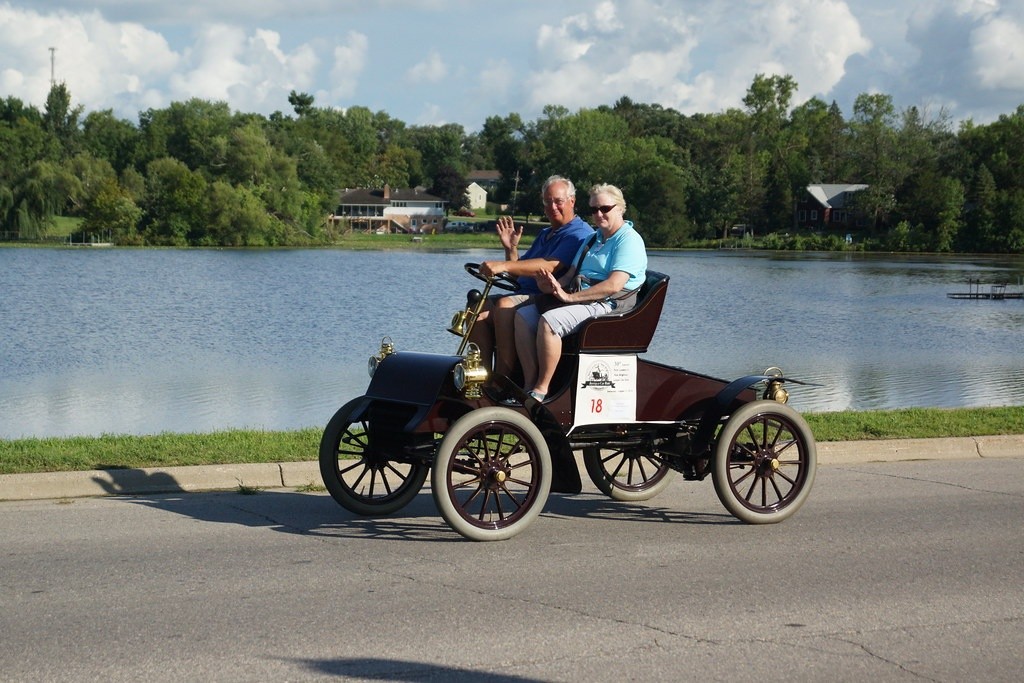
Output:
[568,273,599,305]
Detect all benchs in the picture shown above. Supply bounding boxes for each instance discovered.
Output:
[491,269,670,355]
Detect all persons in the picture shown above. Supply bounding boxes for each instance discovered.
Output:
[466,174,596,400]
[513,182,648,404]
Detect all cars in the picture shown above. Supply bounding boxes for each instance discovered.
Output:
[316,260,819,543]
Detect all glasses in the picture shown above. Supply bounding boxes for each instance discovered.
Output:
[543,196,572,207]
[590,203,617,214]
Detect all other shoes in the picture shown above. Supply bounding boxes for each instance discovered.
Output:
[527,389,544,403]
